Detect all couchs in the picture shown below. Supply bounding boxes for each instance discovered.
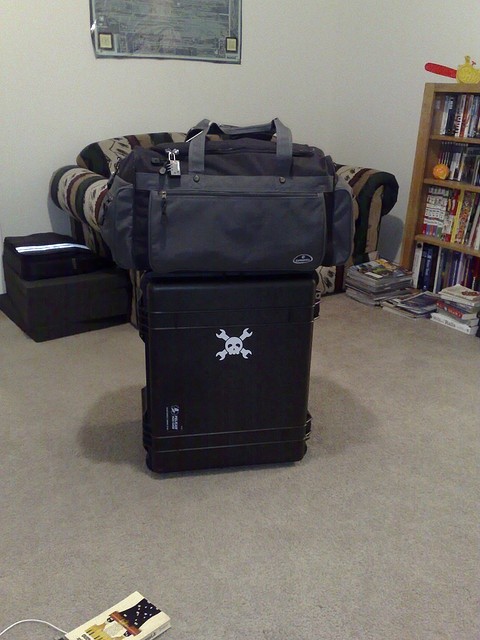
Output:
[48,129,397,292]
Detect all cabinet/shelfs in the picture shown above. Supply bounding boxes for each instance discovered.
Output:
[397,83,478,271]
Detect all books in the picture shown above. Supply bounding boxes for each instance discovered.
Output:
[434,299,478,319]
[381,288,436,321]
[418,186,479,249]
[429,310,480,336]
[439,287,480,307]
[421,244,436,291]
[409,240,423,288]
[432,142,480,185]
[349,258,414,308]
[437,95,479,138]
[438,246,480,301]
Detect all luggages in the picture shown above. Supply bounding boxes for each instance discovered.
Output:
[140,270,321,476]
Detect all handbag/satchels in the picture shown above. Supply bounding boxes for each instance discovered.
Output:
[94,115,358,280]
